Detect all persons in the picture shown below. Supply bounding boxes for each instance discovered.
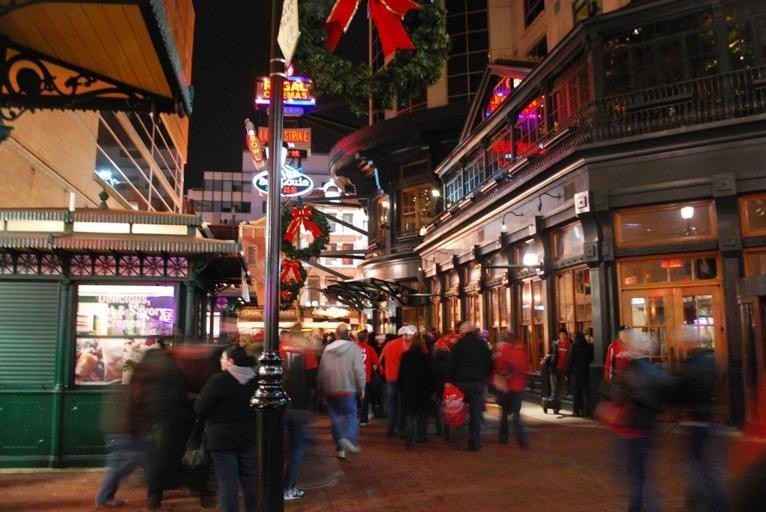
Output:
[96,332,318,511]
[542,329,592,416]
[280,324,532,459]
[603,327,766,512]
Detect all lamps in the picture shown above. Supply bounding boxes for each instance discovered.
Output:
[538,192,560,212]
[680,205,696,235]
[501,210,524,230]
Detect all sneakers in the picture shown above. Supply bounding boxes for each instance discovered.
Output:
[282,487,305,500]
[542,397,549,414]
[334,438,359,459]
[95,497,125,508]
